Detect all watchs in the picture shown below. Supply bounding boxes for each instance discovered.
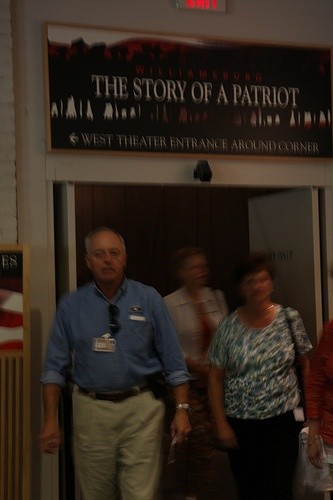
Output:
[175,403,192,415]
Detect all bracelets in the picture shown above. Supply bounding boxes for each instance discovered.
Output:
[307,440,317,446]
[197,300,205,303]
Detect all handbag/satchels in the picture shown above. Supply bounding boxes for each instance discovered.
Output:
[285,308,306,408]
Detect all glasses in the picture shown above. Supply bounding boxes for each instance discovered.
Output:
[108,303,121,334]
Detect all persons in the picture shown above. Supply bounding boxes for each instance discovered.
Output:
[163,246,229,500]
[39,228,192,500]
[206,255,312,500]
[306,319,333,500]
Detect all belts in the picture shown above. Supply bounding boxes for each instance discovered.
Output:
[77,384,152,403]
